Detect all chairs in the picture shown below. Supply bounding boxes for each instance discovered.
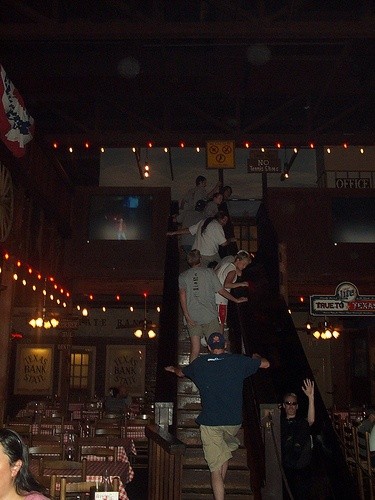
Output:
[124,414,152,469]
[7,399,122,500]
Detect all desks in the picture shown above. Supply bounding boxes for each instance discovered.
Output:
[330,411,362,420]
[10,403,147,500]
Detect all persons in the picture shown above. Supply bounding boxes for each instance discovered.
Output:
[166,210,237,268]
[164,332,270,500]
[214,250,254,353]
[270,378,315,500]
[113,217,126,239]
[0,428,53,500]
[103,385,133,412]
[178,249,248,365]
[202,192,223,218]
[221,186,233,200]
[172,199,208,253]
[181,175,208,211]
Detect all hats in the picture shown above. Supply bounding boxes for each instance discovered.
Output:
[207,333,225,350]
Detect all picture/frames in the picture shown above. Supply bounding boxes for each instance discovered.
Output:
[14,343,56,396]
[105,343,146,397]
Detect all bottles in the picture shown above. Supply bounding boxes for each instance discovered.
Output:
[99,467,113,492]
[64,436,75,461]
[84,417,92,437]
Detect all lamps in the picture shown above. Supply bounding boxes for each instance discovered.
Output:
[312,317,341,340]
[28,276,60,329]
[281,173,289,181]
[134,294,156,339]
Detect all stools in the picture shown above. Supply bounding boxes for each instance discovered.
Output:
[330,400,375,500]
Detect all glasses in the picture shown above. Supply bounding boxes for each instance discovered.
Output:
[284,401,298,405]
